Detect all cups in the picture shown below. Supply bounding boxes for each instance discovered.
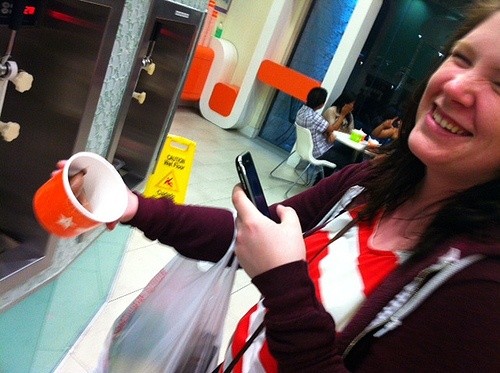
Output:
[32,151,128,239]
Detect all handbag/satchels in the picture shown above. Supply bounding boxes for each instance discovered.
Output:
[94,207,239,373]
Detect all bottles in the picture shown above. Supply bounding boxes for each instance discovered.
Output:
[215,21,224,38]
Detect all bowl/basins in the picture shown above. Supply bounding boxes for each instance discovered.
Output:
[351,131,362,142]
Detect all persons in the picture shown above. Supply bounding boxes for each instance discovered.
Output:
[50,0,500,373]
[295,86,355,188]
[371,116,402,147]
[322,95,355,163]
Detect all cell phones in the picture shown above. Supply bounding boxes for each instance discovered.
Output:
[235,150,271,220]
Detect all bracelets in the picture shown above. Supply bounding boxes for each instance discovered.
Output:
[382,123,386,127]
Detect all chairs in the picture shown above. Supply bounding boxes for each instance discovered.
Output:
[270,122,337,197]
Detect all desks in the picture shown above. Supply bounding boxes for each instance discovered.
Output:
[331,130,383,167]
